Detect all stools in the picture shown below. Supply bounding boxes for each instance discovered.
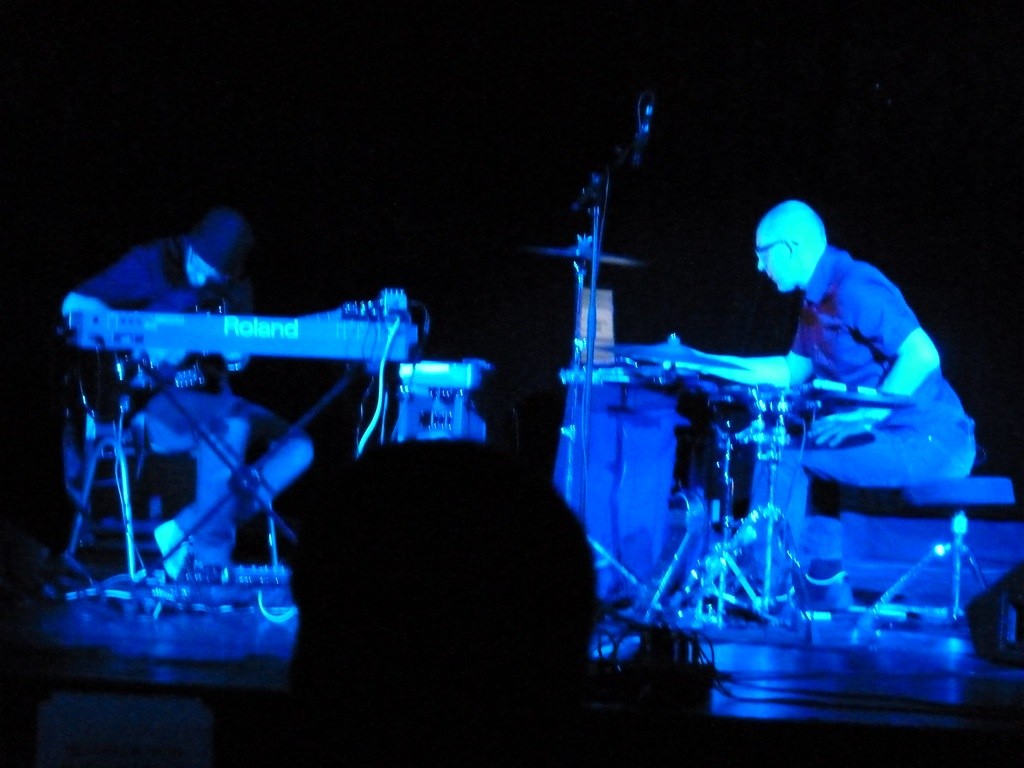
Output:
[55,368,164,561]
[839,476,1017,628]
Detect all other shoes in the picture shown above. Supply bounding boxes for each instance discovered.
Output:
[769,572,856,611]
[724,569,797,605]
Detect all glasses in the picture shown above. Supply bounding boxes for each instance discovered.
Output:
[752,240,794,260]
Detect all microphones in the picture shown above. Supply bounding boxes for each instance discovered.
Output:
[640,96,656,141]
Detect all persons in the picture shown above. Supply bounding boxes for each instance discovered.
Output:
[62,207,316,581]
[259,440,600,768]
[672,199,976,624]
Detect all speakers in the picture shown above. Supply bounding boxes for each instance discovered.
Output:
[965,560,1024,671]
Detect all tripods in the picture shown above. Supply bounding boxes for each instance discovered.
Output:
[648,391,814,629]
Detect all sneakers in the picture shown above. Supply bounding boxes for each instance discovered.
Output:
[153,519,188,579]
